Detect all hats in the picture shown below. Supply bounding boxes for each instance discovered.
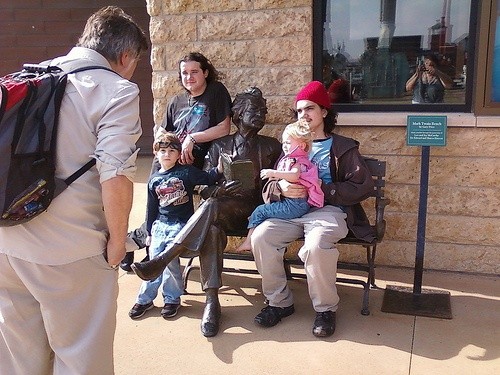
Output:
[295,80,332,110]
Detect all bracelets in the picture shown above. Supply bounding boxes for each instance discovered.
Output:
[188,134,198,144]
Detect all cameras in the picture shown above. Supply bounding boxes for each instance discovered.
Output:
[419,60,429,72]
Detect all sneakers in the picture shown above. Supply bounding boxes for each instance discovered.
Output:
[128,298,155,320]
[119,249,135,272]
[312,311,337,337]
[254,304,295,327]
[160,302,182,319]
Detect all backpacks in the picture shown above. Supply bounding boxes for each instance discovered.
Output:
[0,63,125,231]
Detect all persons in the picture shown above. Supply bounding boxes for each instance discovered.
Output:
[0,5,149,375]
[130,88,283,337]
[119,52,232,271]
[128,134,223,320]
[253,81,375,337]
[405,49,448,104]
[236,118,324,250]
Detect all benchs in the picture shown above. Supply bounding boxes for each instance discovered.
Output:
[180,155,392,316]
